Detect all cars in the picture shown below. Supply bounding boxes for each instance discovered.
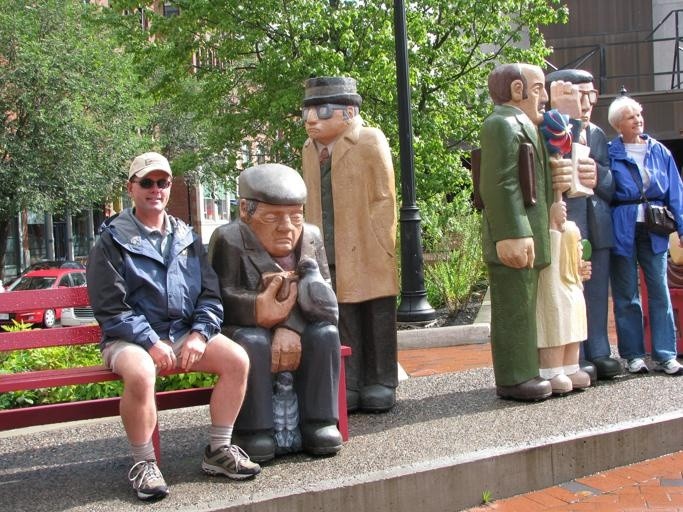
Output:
[0,268,86,328]
[59,282,99,328]
[2,261,86,291]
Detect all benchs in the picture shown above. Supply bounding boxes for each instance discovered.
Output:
[0,272,352,466]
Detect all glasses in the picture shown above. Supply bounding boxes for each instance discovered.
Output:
[133,178,172,189]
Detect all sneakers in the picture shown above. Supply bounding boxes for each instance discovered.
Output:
[653,358,683,375]
[625,358,648,373]
[128,458,169,500]
[201,443,262,480]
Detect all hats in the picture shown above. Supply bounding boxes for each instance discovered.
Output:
[129,152,172,178]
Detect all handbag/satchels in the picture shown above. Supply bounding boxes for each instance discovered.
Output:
[646,204,678,236]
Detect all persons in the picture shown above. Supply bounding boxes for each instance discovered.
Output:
[83,151,262,500]
[298,74,400,418]
[532,201,592,396]
[539,69,620,386]
[210,163,345,466]
[468,63,572,402]
[602,95,683,376]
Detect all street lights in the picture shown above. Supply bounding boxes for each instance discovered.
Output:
[183,172,193,227]
